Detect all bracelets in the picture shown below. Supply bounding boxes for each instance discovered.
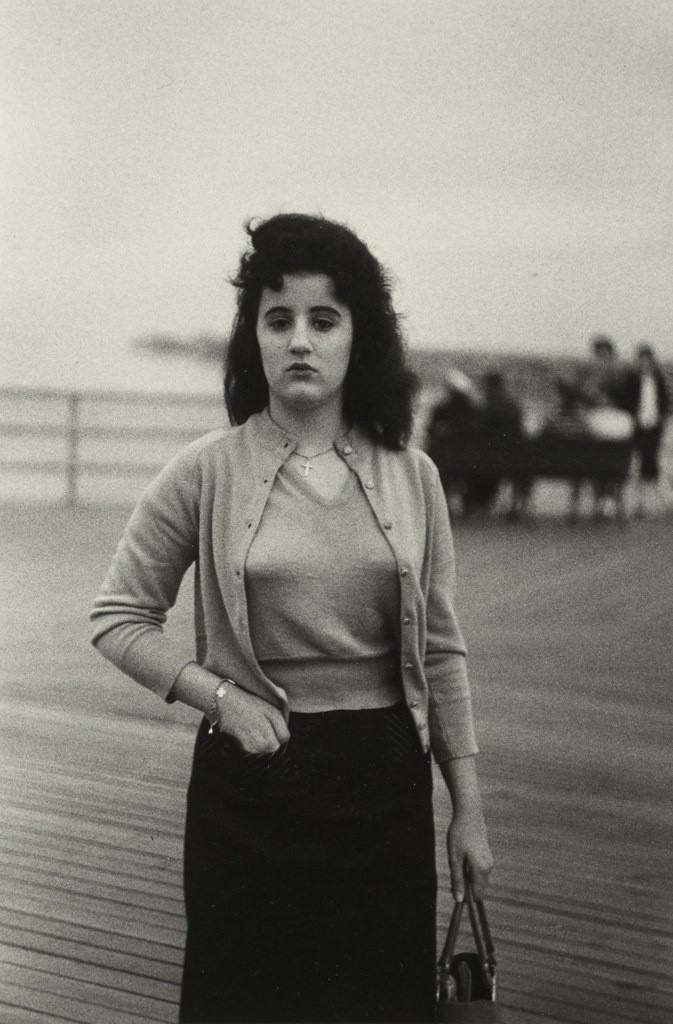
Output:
[206,678,237,735]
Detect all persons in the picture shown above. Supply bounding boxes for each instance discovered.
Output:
[422,340,666,523]
[91,214,494,1024]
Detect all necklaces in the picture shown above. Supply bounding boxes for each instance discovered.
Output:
[291,445,337,476]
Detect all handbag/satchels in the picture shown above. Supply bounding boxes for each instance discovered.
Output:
[436,871,497,1004]
